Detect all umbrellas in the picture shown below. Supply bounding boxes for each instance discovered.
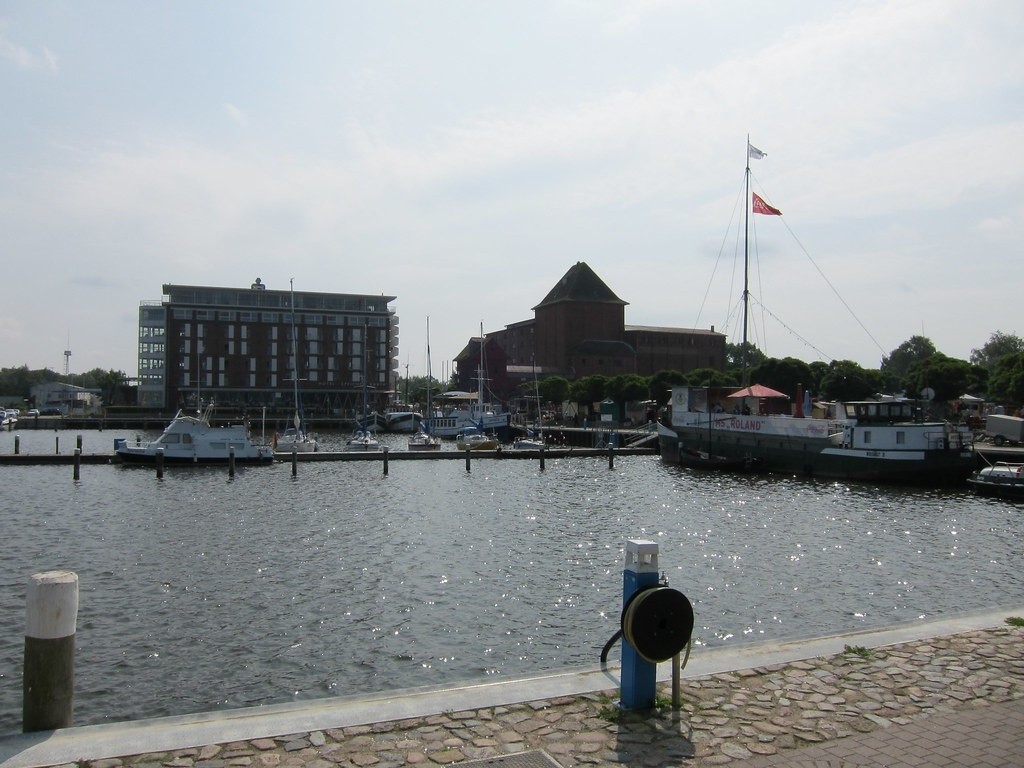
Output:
[726,383,788,416]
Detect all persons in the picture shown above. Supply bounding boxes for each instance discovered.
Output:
[704,389,1024,453]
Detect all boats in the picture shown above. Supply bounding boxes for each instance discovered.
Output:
[116,403,276,466]
[357,406,383,435]
[964,457,1024,497]
[385,406,424,435]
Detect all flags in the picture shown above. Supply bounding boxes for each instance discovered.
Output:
[748,143,768,159]
[752,192,782,216]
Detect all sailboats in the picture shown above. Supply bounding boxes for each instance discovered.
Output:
[455,320,496,450]
[344,317,379,452]
[407,317,441,451]
[651,127,980,494]
[272,278,317,454]
[513,352,543,449]
[422,360,510,438]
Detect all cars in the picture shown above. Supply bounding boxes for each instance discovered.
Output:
[42,407,62,416]
[0,408,20,418]
[28,408,40,417]
